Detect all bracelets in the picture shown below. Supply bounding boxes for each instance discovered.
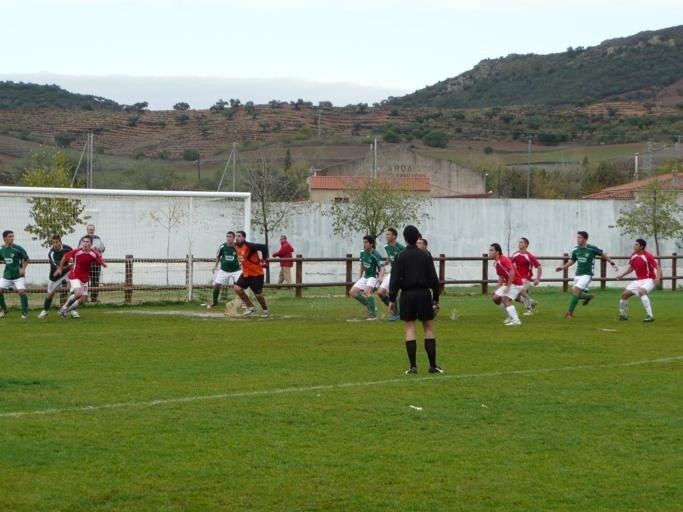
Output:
[610,262,615,266]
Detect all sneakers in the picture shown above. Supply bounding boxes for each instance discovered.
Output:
[261,310,268,317]
[565,312,573,319]
[58,305,80,319]
[0,310,9,317]
[37,309,48,318]
[644,316,655,322]
[207,303,216,309]
[504,300,538,326]
[243,306,257,314]
[429,366,444,374]
[583,293,594,305]
[366,306,400,321]
[405,367,417,375]
[21,311,27,318]
[616,314,627,321]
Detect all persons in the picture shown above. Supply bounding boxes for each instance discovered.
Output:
[272,234,295,289]
[349,228,428,321]
[38,234,82,320]
[555,231,620,319]
[207,232,268,318]
[389,224,445,373]
[0,230,30,320]
[488,237,543,324]
[52,236,108,320]
[614,240,664,325]
[77,222,106,305]
